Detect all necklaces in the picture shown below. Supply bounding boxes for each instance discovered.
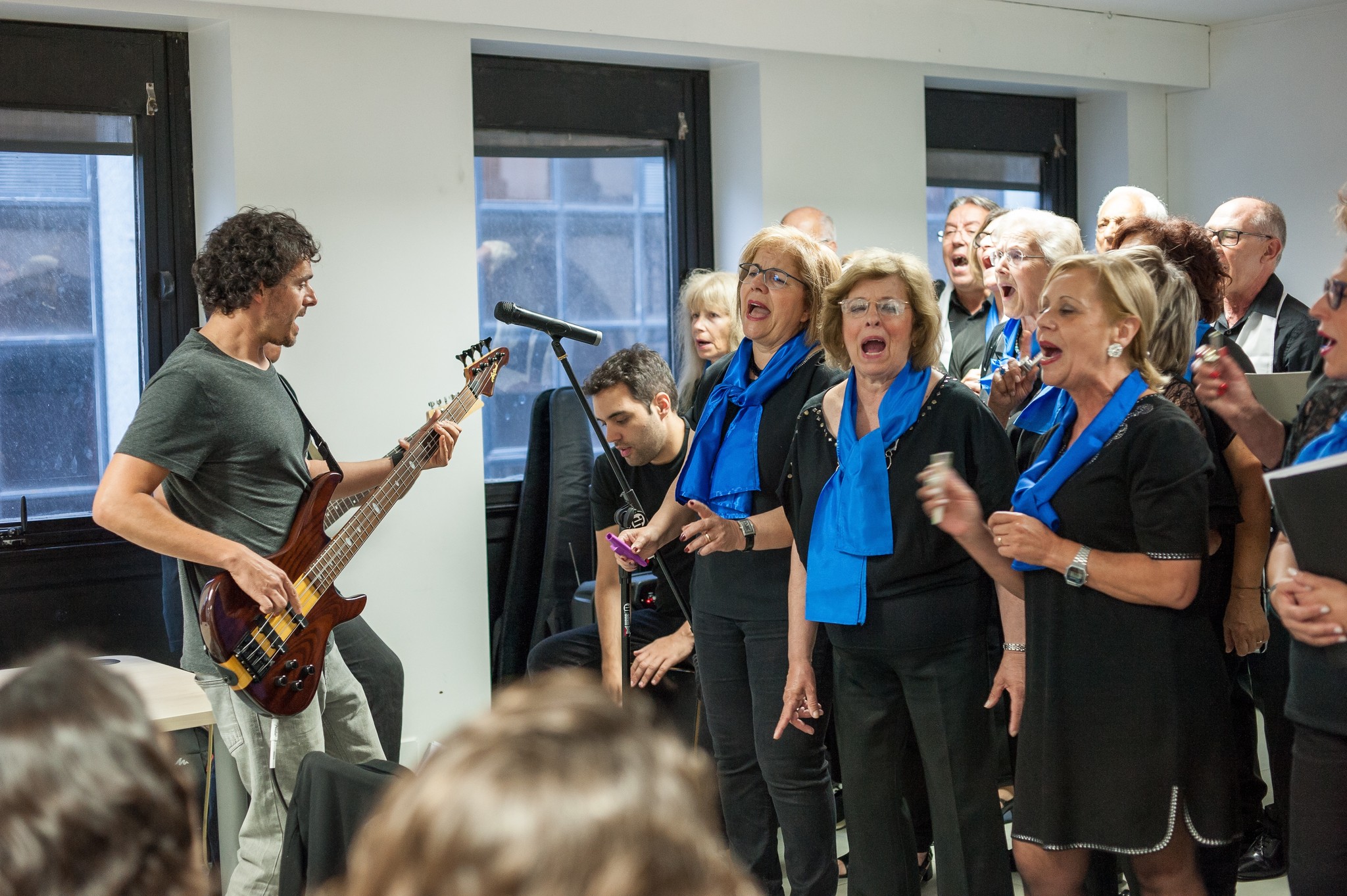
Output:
[748,352,764,377]
[1015,325,1021,354]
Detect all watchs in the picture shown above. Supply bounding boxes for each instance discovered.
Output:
[736,518,756,553]
[1064,543,1093,587]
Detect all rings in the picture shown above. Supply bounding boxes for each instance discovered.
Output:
[706,533,711,542]
[997,535,1004,546]
[1256,640,1263,643]
[1000,368,1007,376]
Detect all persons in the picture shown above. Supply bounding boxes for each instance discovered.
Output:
[306,664,765,896]
[1265,252,1347,896]
[771,246,1028,896]
[912,250,1214,896]
[666,267,745,433]
[602,224,847,896]
[525,344,695,740]
[778,184,1326,883]
[91,207,463,896]
[0,640,212,896]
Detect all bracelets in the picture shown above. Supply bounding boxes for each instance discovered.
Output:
[1267,583,1281,619]
[1230,585,1265,593]
[1003,643,1026,651]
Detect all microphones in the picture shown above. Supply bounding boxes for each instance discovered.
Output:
[493,301,603,347]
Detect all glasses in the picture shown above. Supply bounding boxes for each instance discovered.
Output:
[988,250,1044,268]
[1322,277,1347,310]
[736,261,806,290]
[973,231,990,248]
[939,229,976,243]
[839,299,910,318]
[1201,226,1271,247]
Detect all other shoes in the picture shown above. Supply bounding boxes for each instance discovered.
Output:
[1237,811,1287,880]
[837,852,852,878]
[916,846,934,887]
[835,789,848,827]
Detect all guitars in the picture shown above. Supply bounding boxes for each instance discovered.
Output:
[200,333,510,716]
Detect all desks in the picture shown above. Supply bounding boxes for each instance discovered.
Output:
[1,656,246,896]
